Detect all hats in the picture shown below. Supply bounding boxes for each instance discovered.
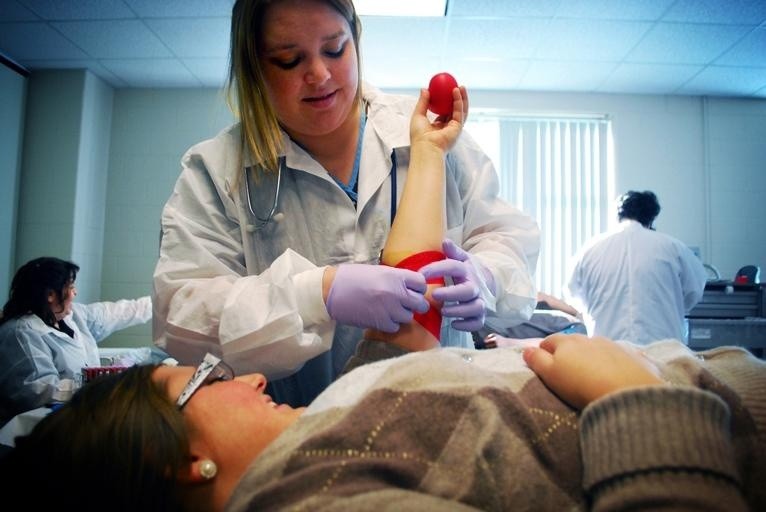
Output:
[620,190,661,226]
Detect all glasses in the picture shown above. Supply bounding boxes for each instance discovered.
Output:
[175,353,234,410]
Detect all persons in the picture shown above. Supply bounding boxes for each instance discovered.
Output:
[150,0,545,413]
[12,87,766,512]
[0,256,170,426]
[564,186,707,346]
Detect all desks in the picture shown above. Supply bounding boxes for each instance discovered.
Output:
[683,319,766,357]
